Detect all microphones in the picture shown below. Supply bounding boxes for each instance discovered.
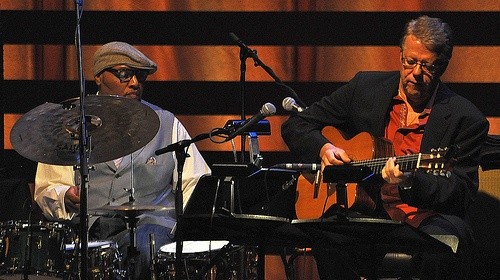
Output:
[224,103,276,140]
[282,97,305,114]
[278,163,321,169]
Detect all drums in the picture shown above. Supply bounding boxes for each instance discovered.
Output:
[66,239,124,280]
[0,217,72,280]
[149,239,263,280]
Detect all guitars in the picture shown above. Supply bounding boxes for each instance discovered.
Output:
[295,126,461,220]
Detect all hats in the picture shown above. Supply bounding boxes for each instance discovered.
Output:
[92,41,157,78]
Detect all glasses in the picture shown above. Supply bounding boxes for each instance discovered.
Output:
[104,67,149,83]
[400,49,435,71]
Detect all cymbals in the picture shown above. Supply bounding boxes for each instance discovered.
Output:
[88,200,177,219]
[9,94,161,166]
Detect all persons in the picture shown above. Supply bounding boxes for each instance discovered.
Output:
[280,17,489,280]
[34,41,211,280]
